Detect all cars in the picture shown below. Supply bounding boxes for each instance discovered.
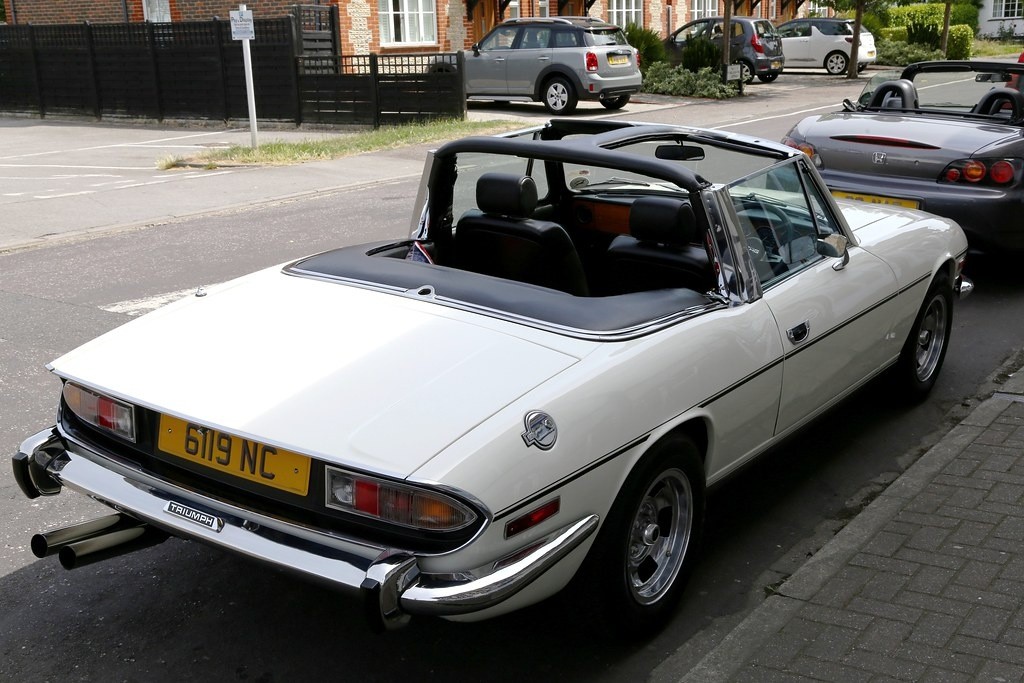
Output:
[776,17,876,76]
[657,16,785,85]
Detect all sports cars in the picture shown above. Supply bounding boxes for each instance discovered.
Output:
[5,114,970,647]
[764,60,1024,265]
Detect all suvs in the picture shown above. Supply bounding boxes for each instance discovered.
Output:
[425,15,642,116]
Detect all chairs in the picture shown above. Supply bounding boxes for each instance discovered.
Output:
[883,79,921,110]
[556,32,576,46]
[713,25,723,39]
[969,85,1024,121]
[453,171,590,296]
[525,30,541,48]
[601,195,717,296]
